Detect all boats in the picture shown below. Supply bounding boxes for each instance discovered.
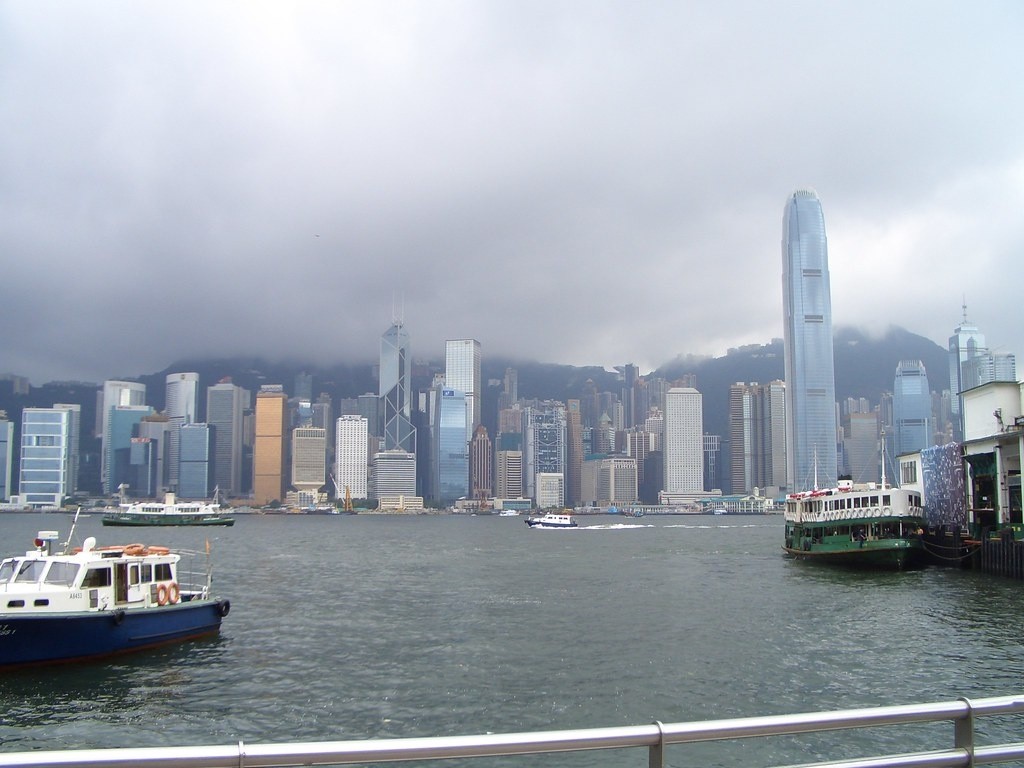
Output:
[713,508,728,515]
[499,510,520,516]
[781,420,928,572]
[0,503,232,677]
[102,483,235,527]
[623,511,644,517]
[324,508,340,514]
[523,512,579,528]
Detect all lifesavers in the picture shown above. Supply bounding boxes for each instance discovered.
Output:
[786,513,818,522]
[156,584,168,607]
[858,508,865,518]
[826,511,830,521]
[786,537,793,548]
[851,509,858,519]
[830,511,835,521]
[802,539,812,551]
[73,543,170,557]
[835,510,840,520]
[840,510,846,520]
[822,512,826,521]
[873,507,882,517]
[168,583,180,604]
[882,507,893,517]
[865,508,873,518]
[115,610,125,626]
[217,599,231,617]
[846,509,851,519]
[908,506,924,519]
